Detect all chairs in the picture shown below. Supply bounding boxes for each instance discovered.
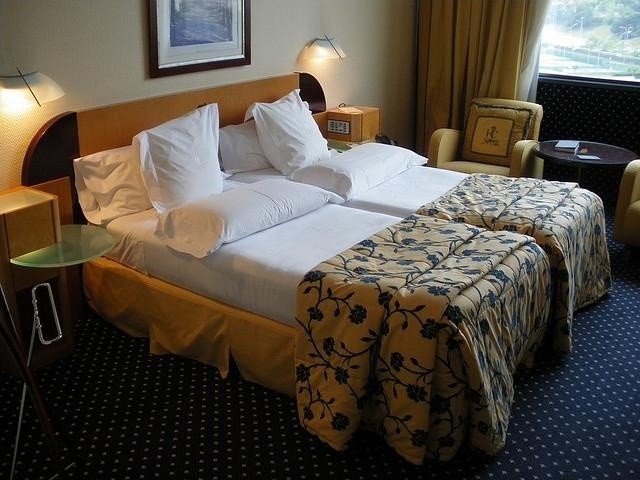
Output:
[613,160,640,247]
[424,97,545,179]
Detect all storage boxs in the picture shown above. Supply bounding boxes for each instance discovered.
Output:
[326,105,380,142]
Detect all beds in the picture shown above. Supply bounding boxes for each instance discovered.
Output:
[215,73,617,353]
[74,91,552,469]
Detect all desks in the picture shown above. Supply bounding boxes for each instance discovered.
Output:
[321,141,377,153]
[533,139,640,196]
[9,223,116,268]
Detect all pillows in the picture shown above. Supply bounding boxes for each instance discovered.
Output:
[290,140,431,202]
[72,145,153,225]
[132,102,231,213]
[218,101,314,174]
[153,181,346,260]
[245,88,328,175]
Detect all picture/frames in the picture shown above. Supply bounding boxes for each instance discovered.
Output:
[145,0,251,78]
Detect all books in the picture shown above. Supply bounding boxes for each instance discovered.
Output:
[555,140,580,153]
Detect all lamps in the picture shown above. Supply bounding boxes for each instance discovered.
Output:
[0,72,66,109]
[303,38,346,60]
